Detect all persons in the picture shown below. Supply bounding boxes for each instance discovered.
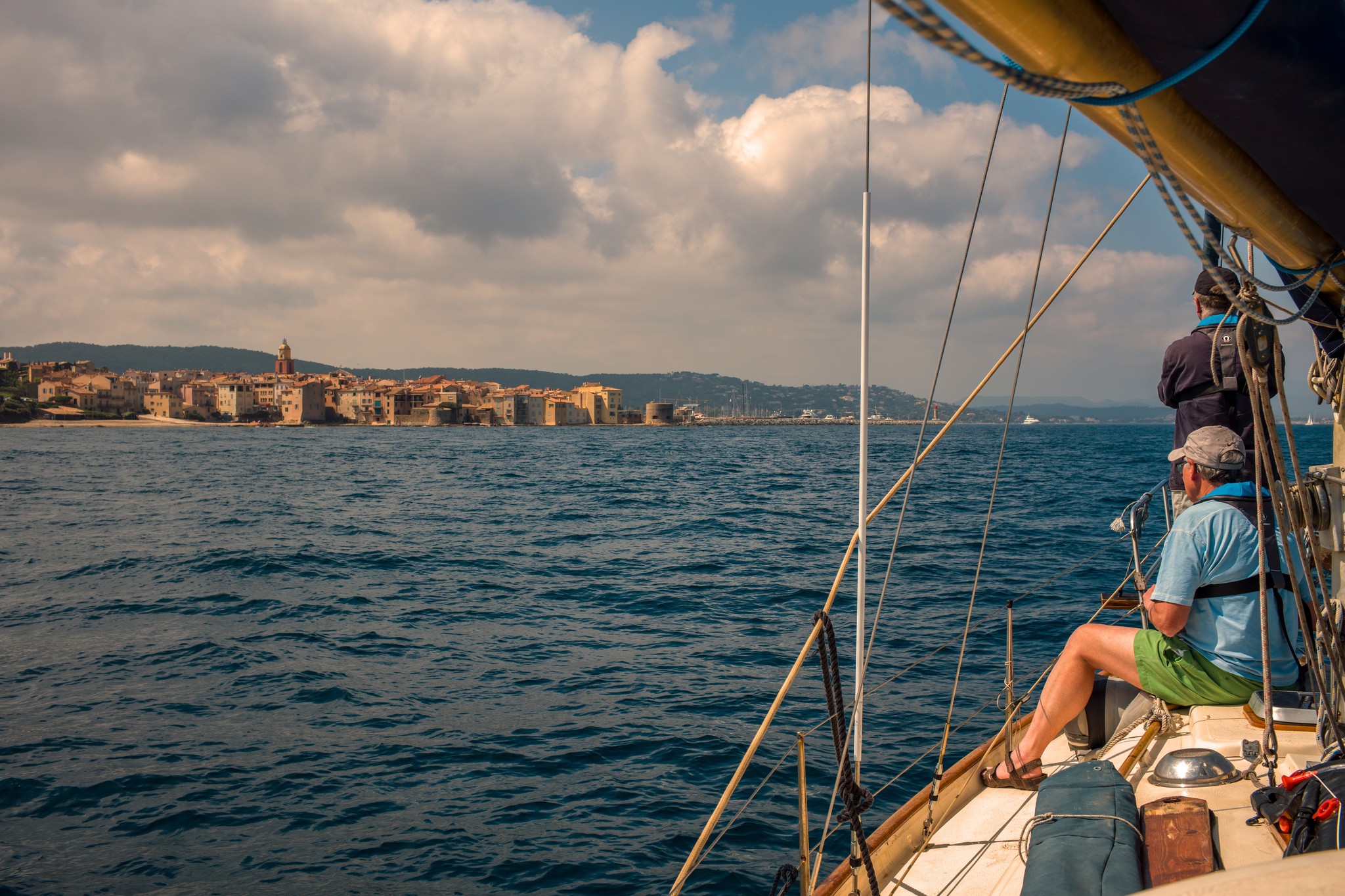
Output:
[1158,266,1285,516]
[978,425,1317,791]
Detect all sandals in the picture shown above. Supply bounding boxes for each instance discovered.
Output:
[981,750,1047,791]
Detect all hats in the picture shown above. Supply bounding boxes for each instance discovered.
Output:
[1167,426,1246,470]
[1192,266,1241,297]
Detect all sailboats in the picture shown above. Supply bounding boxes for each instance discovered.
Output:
[1305,414,1314,425]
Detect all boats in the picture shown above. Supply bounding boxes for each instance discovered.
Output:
[824,413,837,419]
[885,413,894,420]
[1023,413,1040,424]
[840,412,855,419]
[799,406,821,419]
[770,409,791,418]
[867,407,885,420]
